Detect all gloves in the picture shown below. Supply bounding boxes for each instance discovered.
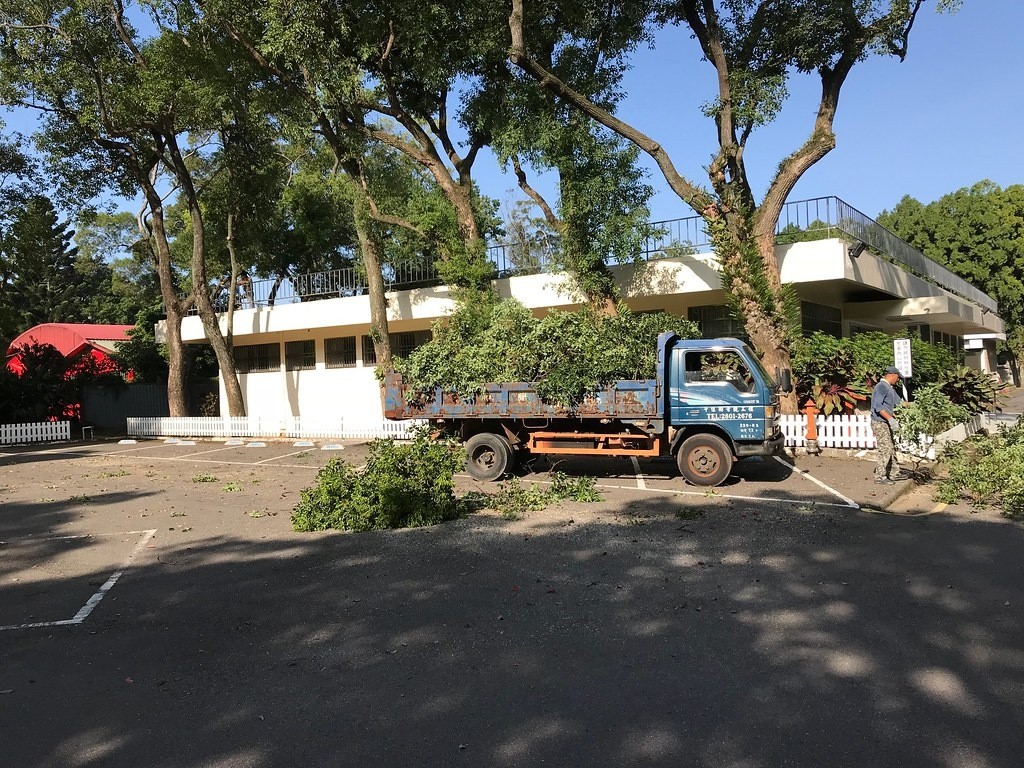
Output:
[888,417,900,432]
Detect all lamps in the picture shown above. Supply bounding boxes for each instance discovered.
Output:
[849,240,869,258]
[982,307,990,314]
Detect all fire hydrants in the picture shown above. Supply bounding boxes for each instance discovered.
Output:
[800,399,823,456]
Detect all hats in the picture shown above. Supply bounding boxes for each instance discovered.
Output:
[885,366,904,379]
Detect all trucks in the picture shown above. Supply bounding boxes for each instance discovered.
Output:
[381,330,787,488]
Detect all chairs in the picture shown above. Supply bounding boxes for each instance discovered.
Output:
[687,359,703,381]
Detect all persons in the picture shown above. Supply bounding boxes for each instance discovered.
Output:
[870,366,917,485]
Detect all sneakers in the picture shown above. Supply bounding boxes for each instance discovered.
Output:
[889,471,908,480]
[875,475,895,485]
